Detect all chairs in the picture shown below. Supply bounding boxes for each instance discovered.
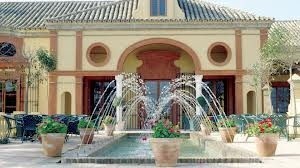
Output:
[2,115,38,144]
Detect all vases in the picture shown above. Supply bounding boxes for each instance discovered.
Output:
[219,126,237,144]
[254,134,280,155]
[151,137,179,167]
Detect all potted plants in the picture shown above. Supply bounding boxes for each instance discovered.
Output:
[201,119,212,135]
[39,119,67,157]
[102,115,117,135]
[78,118,96,145]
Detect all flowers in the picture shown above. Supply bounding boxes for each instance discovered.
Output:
[153,120,181,138]
[217,118,237,128]
[245,118,282,137]
